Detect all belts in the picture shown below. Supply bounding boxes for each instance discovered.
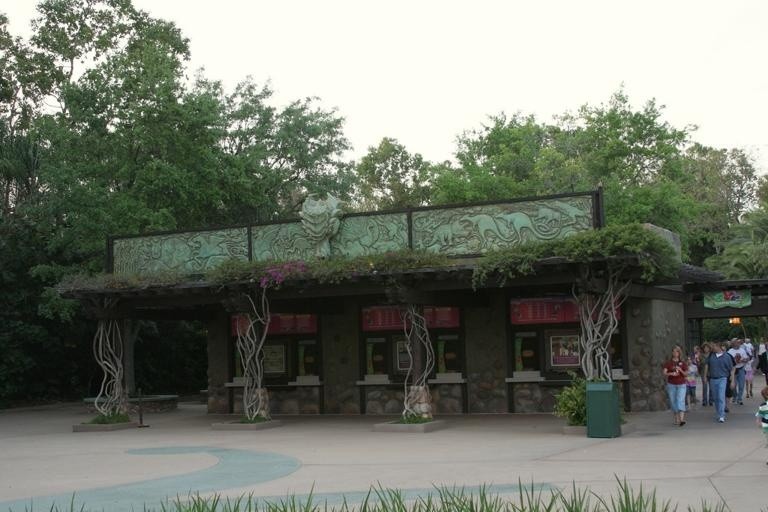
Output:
[712,376,724,379]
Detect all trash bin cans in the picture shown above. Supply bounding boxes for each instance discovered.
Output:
[585,381,621,438]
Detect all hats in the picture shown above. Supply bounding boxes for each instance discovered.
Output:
[701,341,713,352]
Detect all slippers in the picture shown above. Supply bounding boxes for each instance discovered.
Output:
[673,404,691,426]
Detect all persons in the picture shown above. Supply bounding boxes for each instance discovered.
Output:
[659,333,768,426]
[754,386,768,466]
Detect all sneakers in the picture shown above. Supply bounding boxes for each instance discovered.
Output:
[702,391,753,423]
